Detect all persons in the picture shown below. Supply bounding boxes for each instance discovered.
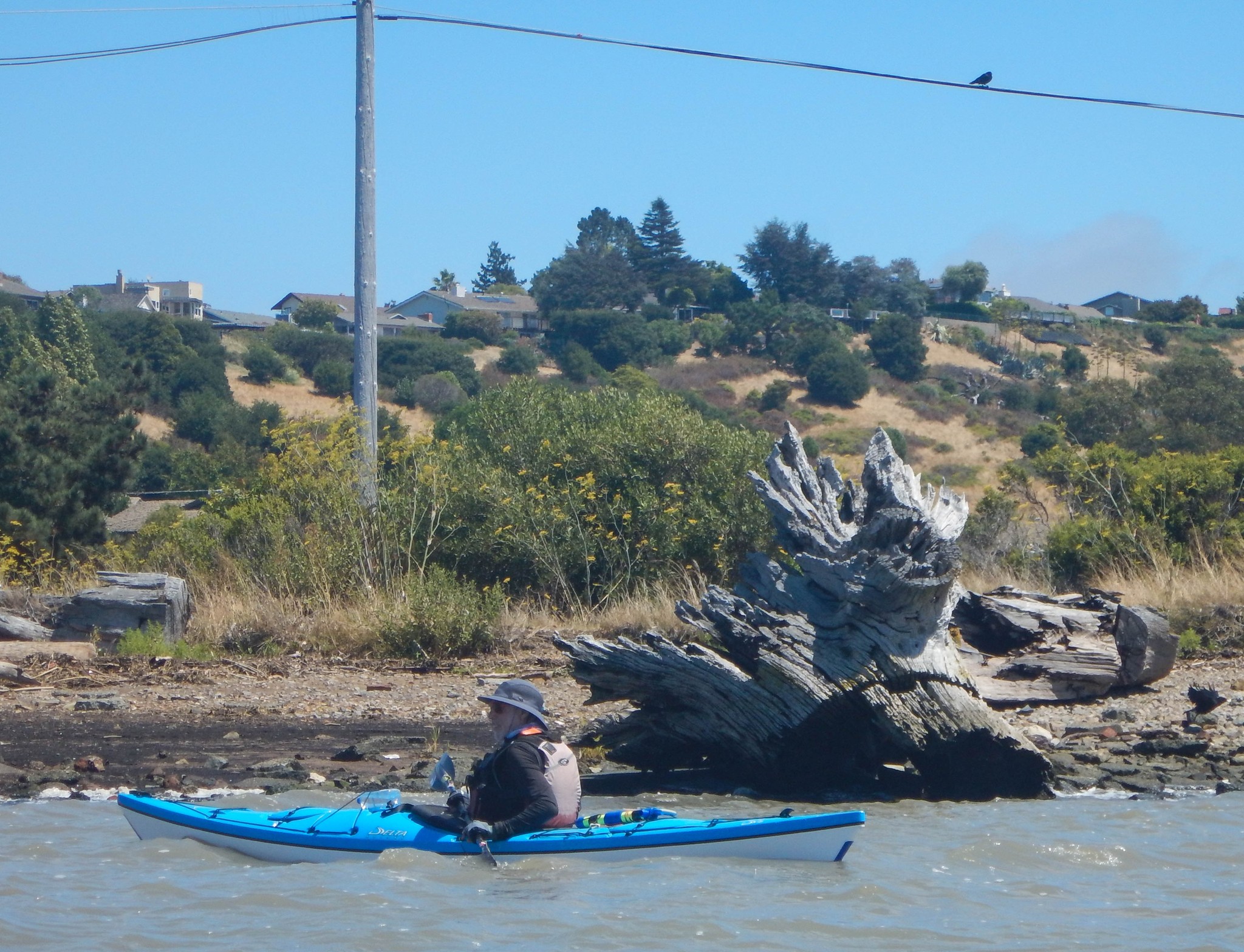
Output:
[447,679,581,843]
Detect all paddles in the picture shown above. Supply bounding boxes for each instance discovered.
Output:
[429,751,498,869]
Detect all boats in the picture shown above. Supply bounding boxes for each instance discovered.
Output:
[114,786,866,866]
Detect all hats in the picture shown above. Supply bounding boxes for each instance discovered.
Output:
[477,679,548,731]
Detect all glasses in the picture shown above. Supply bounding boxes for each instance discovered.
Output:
[488,703,510,714]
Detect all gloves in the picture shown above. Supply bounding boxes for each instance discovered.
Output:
[446,789,470,808]
[461,820,493,842]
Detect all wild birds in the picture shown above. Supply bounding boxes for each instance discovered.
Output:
[969,72,993,88]
